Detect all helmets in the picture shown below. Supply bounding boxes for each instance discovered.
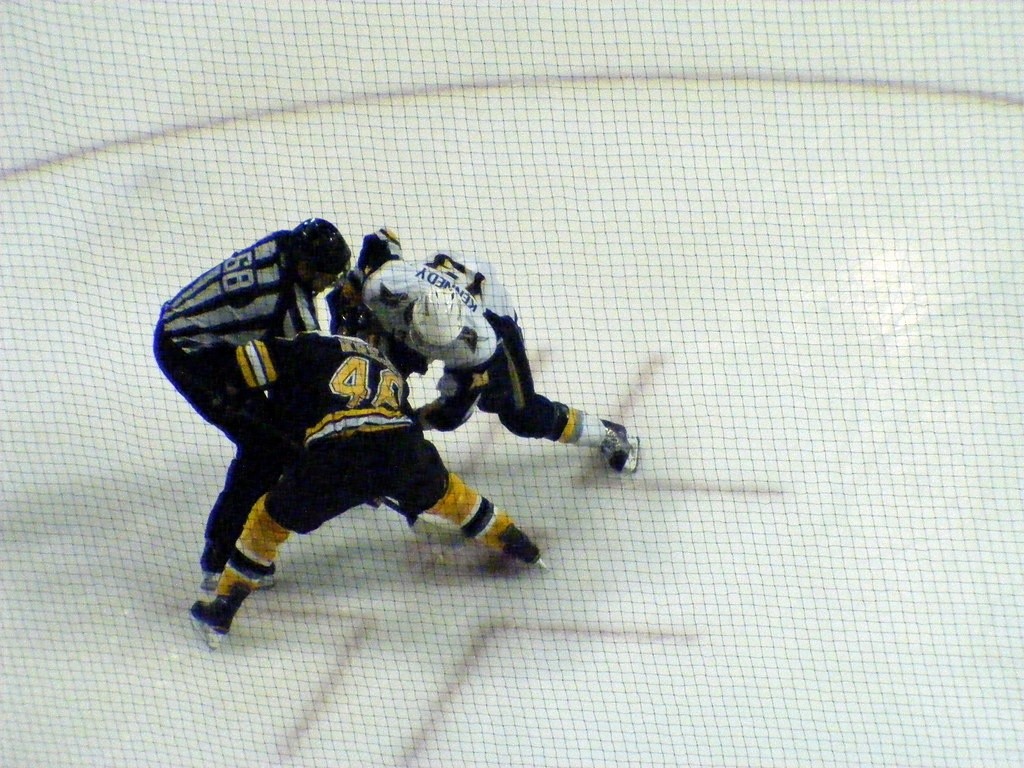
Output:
[287,217,352,275]
[412,292,466,346]
[333,307,381,335]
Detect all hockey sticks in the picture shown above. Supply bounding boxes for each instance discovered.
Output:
[384,497,464,535]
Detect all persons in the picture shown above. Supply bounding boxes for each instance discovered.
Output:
[154,218,640,649]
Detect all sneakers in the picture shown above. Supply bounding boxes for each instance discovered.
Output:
[199,540,276,595]
[598,418,640,472]
[189,581,252,650]
[499,522,548,570]
